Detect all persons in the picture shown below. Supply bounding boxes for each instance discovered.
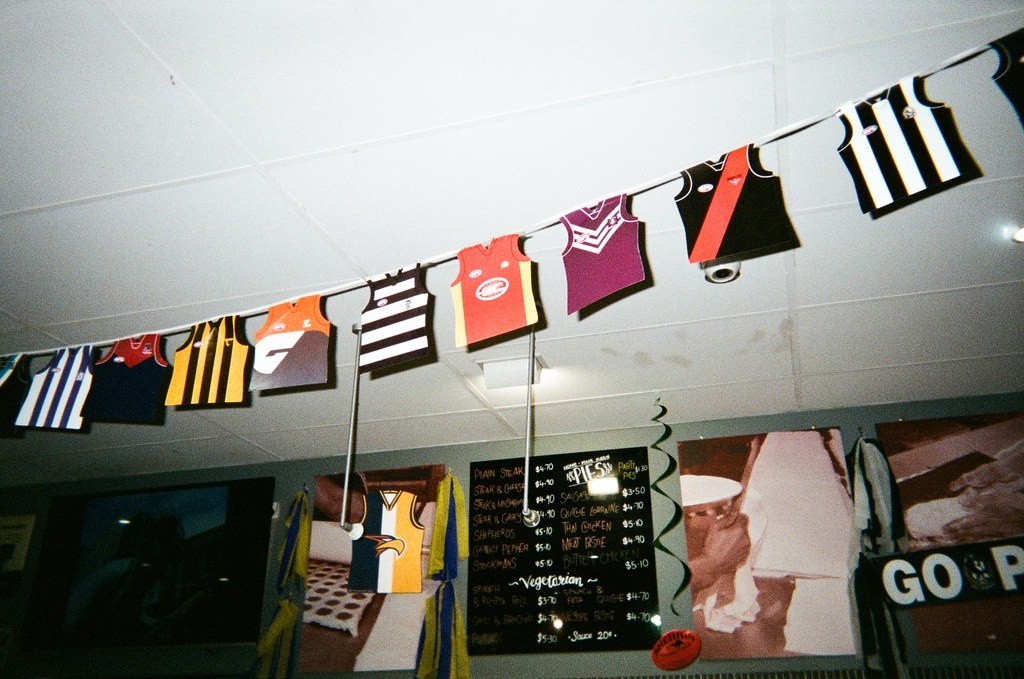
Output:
[943,447,1023,544]
[685,502,749,590]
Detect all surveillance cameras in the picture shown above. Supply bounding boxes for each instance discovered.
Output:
[702,256,741,283]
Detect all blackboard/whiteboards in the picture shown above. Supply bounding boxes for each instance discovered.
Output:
[467,446,661,657]
